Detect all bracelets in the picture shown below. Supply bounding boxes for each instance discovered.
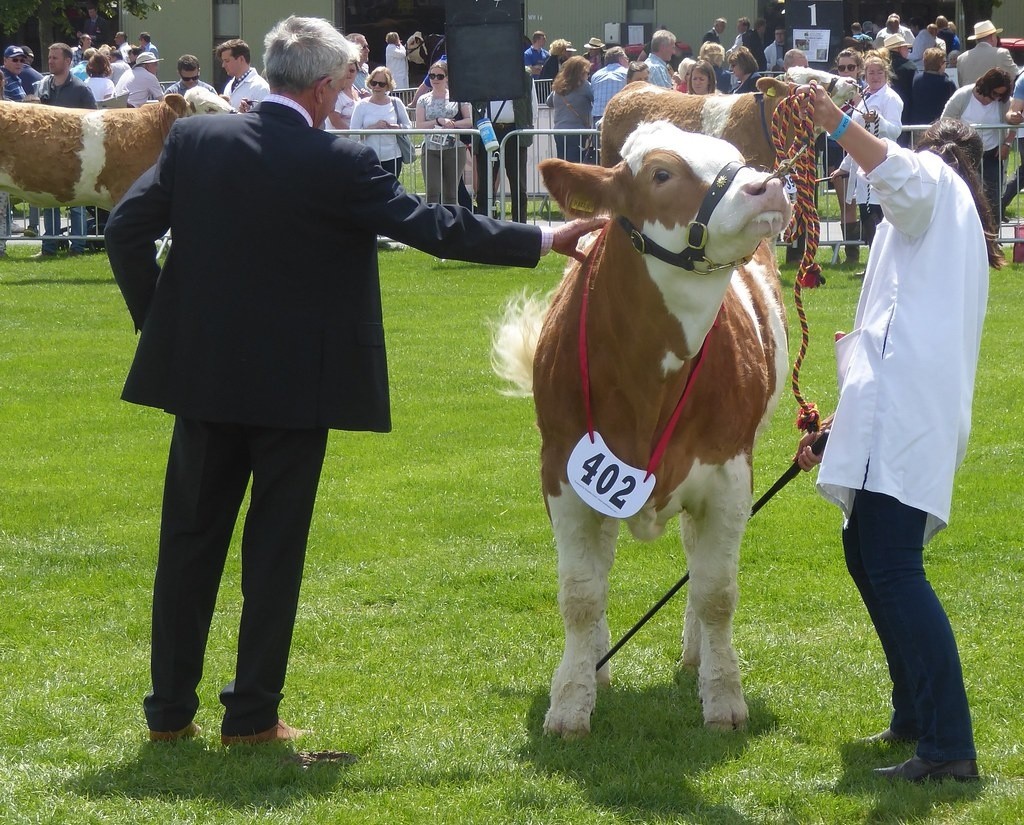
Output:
[827,113,850,141]
[436,117,442,126]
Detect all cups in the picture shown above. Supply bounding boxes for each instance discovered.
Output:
[476,118,499,152]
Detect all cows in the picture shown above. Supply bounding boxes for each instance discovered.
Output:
[600,66,858,278]
[491,119,795,740]
[0,86,238,238]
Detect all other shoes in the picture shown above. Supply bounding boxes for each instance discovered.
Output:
[149,721,201,744]
[220,717,314,748]
[9,205,107,258]
[779,205,1013,268]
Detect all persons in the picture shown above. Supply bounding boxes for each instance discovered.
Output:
[105,15,609,743]
[0,0,1024,264]
[788,76,1003,784]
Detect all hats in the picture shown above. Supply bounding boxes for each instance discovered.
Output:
[565,41,578,52]
[584,37,605,49]
[966,20,1003,40]
[3,45,25,58]
[131,51,165,68]
[883,34,913,49]
[20,46,35,60]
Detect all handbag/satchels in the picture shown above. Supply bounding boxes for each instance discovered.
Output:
[391,96,417,164]
[454,102,472,144]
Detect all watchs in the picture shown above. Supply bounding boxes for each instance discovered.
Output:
[1003,142,1012,148]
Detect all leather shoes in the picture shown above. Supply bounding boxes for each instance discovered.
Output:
[859,725,915,746]
[872,755,980,785]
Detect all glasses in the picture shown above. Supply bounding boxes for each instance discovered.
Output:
[8,56,26,64]
[992,91,1004,98]
[370,79,388,88]
[428,74,448,80]
[348,68,358,74]
[838,64,856,72]
[179,71,199,82]
